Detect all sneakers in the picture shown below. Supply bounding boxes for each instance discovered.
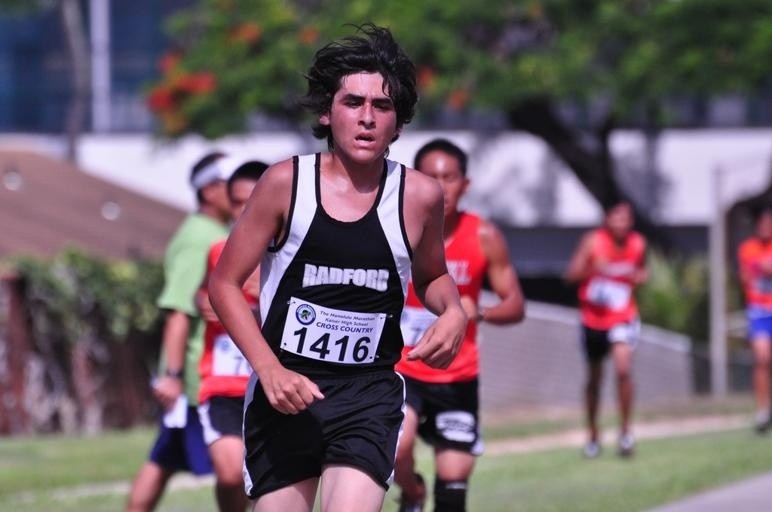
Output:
[618,436,636,452]
[582,440,601,456]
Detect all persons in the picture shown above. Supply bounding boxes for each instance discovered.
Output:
[560,196,648,458]
[193,161,270,512]
[127,153,239,512]
[208,22,469,512]
[737,199,772,434]
[394,139,526,512]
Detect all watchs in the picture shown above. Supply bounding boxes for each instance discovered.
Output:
[478,305,485,319]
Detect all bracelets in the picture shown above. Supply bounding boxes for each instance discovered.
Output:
[166,369,183,378]
